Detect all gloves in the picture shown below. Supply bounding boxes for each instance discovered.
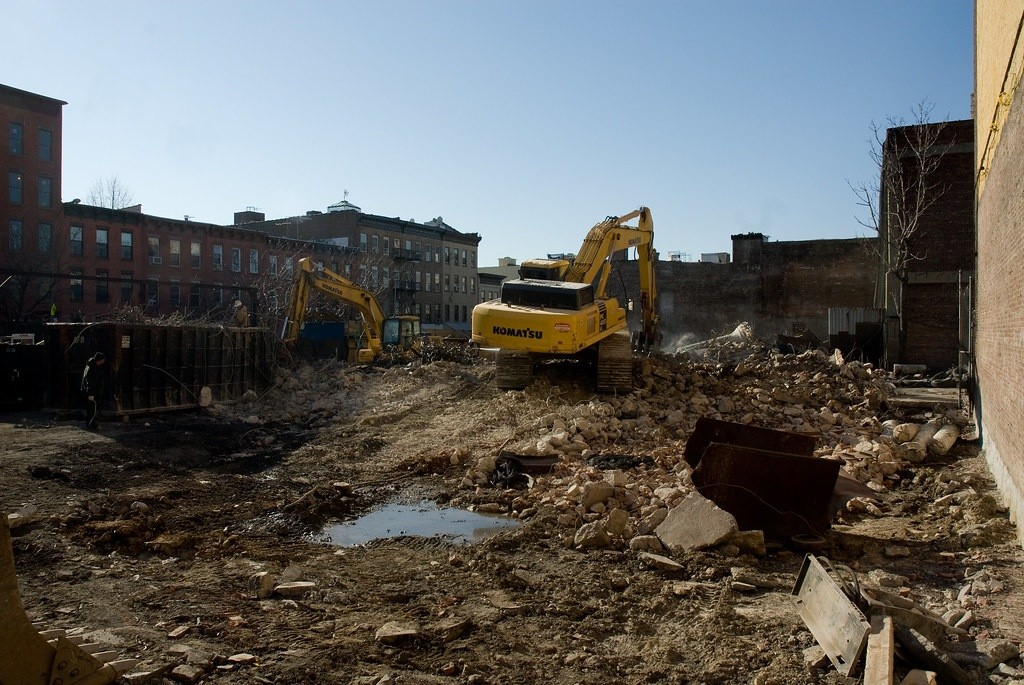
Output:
[89,395,94,401]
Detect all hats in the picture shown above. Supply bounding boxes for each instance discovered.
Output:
[233,300,242,307]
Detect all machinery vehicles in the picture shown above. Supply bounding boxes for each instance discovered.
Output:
[0,509,117,685]
[280,256,443,368]
[468,205,664,396]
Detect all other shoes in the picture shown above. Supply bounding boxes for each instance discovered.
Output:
[90,425,101,430]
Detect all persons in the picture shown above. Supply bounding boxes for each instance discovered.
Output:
[82,352,105,429]
[232,300,248,327]
